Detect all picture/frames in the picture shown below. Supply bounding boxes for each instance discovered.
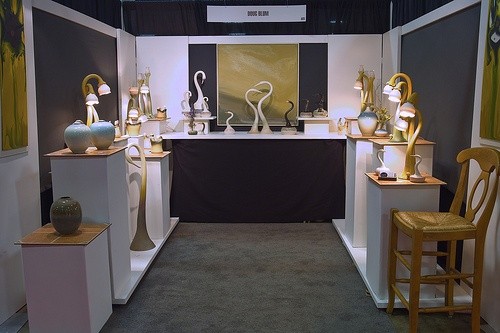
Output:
[216,43,300,126]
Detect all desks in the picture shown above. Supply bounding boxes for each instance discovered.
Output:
[156,128,347,224]
[362,164,447,298]
[130,151,174,238]
[111,135,128,148]
[344,115,384,134]
[181,116,217,134]
[125,130,147,155]
[12,222,116,333]
[43,146,143,300]
[296,116,334,134]
[136,117,172,145]
[344,129,391,248]
[367,135,438,178]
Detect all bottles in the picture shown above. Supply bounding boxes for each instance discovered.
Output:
[50,196,82,236]
[357,103,380,137]
[90,119,116,150]
[64,119,92,154]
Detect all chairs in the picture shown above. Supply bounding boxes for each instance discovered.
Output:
[386,146,500,333]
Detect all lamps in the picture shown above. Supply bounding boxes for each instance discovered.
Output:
[394,90,425,178]
[352,68,380,110]
[79,71,113,126]
[384,70,414,143]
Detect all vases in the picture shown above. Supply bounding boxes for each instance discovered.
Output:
[62,120,93,154]
[90,119,117,150]
[358,112,379,136]
[48,195,82,236]
